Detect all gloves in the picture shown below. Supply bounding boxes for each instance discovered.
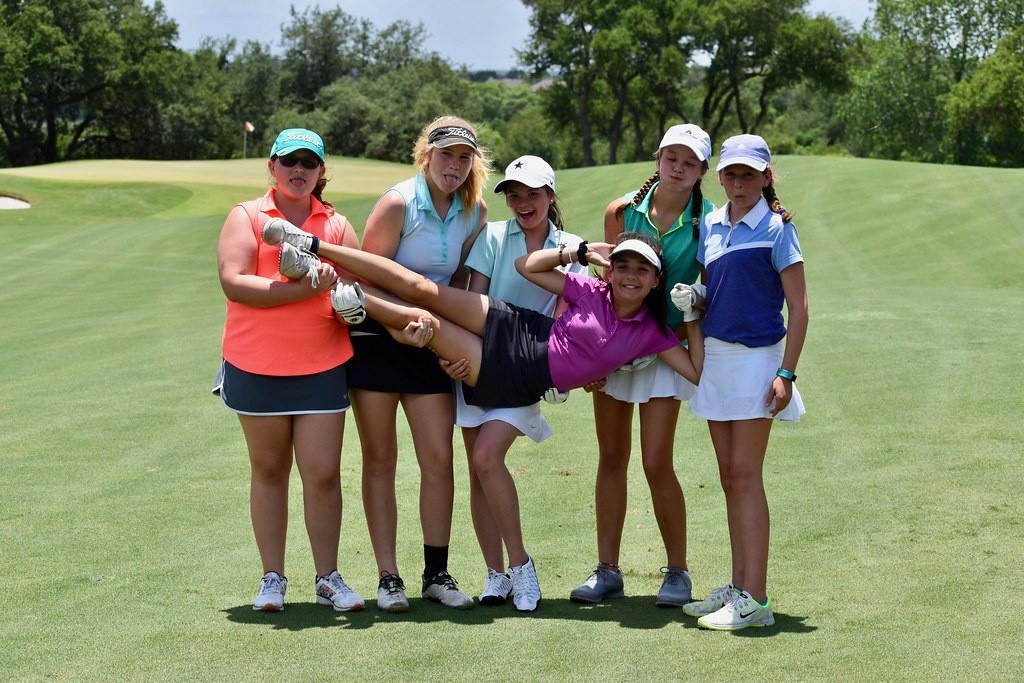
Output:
[684,284,707,322]
[330,282,366,324]
[614,352,662,373]
[540,387,569,404]
[670,283,697,314]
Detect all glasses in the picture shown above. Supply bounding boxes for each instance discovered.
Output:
[273,153,322,170]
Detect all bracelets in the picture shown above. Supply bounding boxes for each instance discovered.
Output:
[568,248,574,264]
[776,368,796,382]
[558,242,566,267]
[577,240,589,266]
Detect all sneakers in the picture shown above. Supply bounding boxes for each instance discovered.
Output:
[261,217,313,251]
[683,583,742,617]
[508,555,542,611]
[277,241,322,290]
[252,571,288,611]
[315,570,366,611]
[697,590,775,632]
[422,570,474,607]
[569,564,624,603]
[656,567,693,606]
[377,570,409,611]
[479,567,513,605]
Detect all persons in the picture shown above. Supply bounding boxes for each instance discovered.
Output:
[211,129,365,610]
[669,132,807,629]
[455,154,587,614]
[264,217,706,409]
[350,116,486,612]
[569,124,718,607]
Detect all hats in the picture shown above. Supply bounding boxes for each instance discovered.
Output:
[494,154,556,195]
[660,124,712,161]
[609,239,661,273]
[428,125,482,158]
[716,133,771,172]
[270,128,325,164]
